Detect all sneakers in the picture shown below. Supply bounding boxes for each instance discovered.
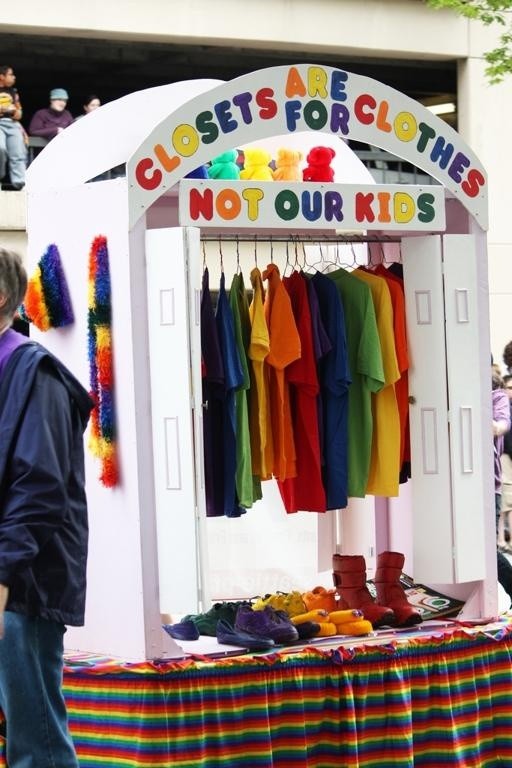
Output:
[163,551,423,651]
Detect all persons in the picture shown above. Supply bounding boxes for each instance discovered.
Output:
[0,63,100,188]
[490,340,512,599]
[0,246,96,768]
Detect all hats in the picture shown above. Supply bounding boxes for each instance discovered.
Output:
[17,244,74,332]
[50,89,69,100]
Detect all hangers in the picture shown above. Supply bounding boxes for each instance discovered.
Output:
[199,229,395,288]
